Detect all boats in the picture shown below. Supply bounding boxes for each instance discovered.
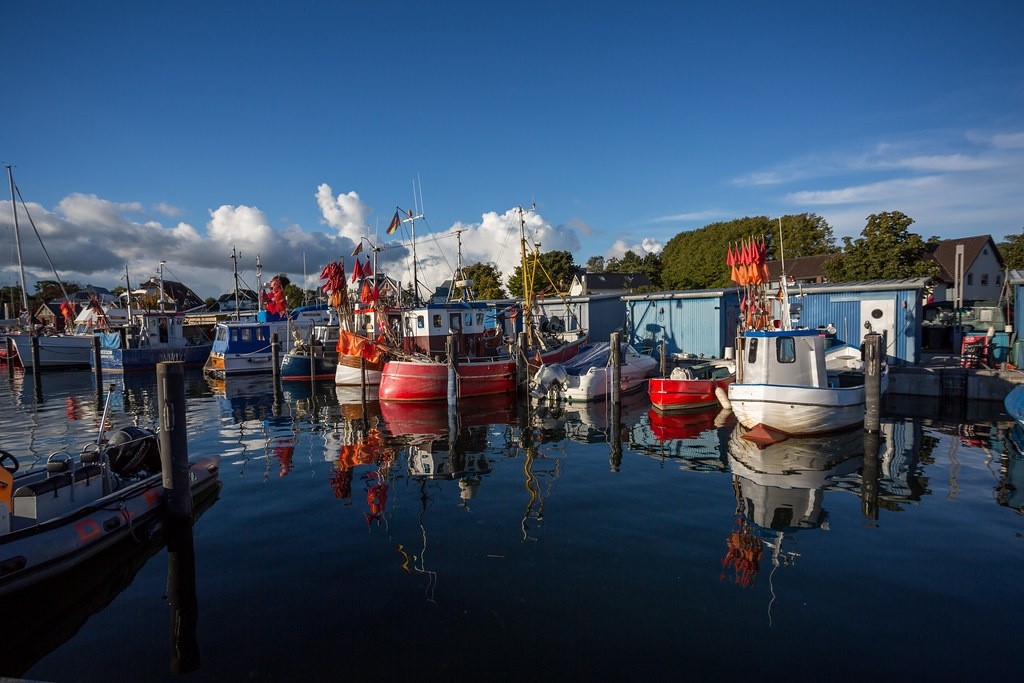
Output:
[204,374,275,426]
[0,382,224,597]
[14,368,96,407]
[331,333,422,385]
[725,214,892,437]
[89,261,217,373]
[377,402,524,438]
[201,320,288,375]
[528,338,660,405]
[281,381,336,403]
[647,414,732,469]
[646,367,731,412]
[280,325,343,381]
[335,386,383,419]
[719,420,889,627]
[377,353,547,403]
[8,332,99,368]
